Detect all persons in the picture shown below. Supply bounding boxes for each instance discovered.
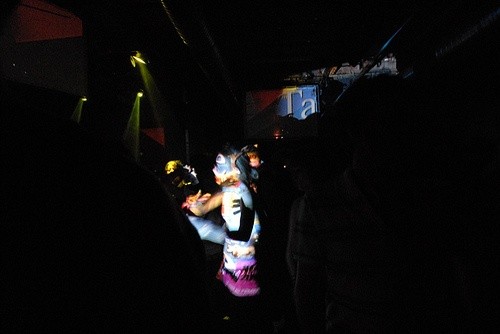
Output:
[283,150,403,333]
[186,149,261,311]
